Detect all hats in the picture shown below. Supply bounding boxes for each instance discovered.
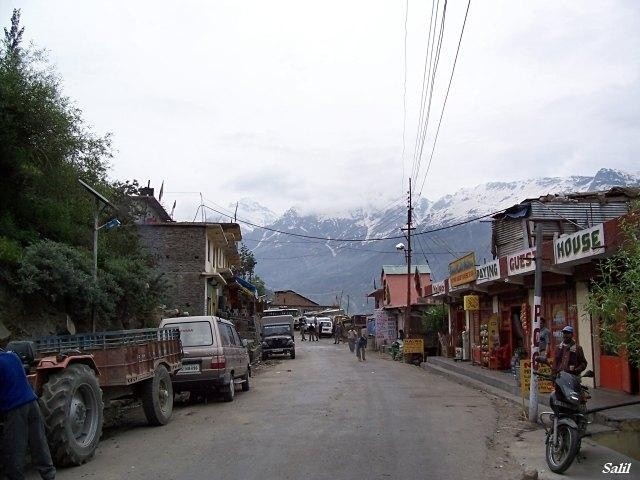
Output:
[561,326,572,333]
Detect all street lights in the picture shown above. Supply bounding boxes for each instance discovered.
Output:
[78,178,121,333]
[396,242,411,336]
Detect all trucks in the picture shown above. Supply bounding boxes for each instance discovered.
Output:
[259,315,296,359]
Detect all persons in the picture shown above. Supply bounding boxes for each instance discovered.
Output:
[0,348,56,480]
[298,316,324,342]
[333,323,343,344]
[552,326,587,375]
[348,327,358,352]
[357,333,367,362]
[539,317,552,356]
[398,329,406,341]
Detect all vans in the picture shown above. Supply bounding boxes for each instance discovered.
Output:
[157,315,252,401]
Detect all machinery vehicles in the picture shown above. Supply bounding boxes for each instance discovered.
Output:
[7,327,185,468]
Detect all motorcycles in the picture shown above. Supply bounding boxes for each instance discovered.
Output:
[532,355,595,474]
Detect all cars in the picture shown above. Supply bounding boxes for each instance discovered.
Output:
[303,315,333,337]
